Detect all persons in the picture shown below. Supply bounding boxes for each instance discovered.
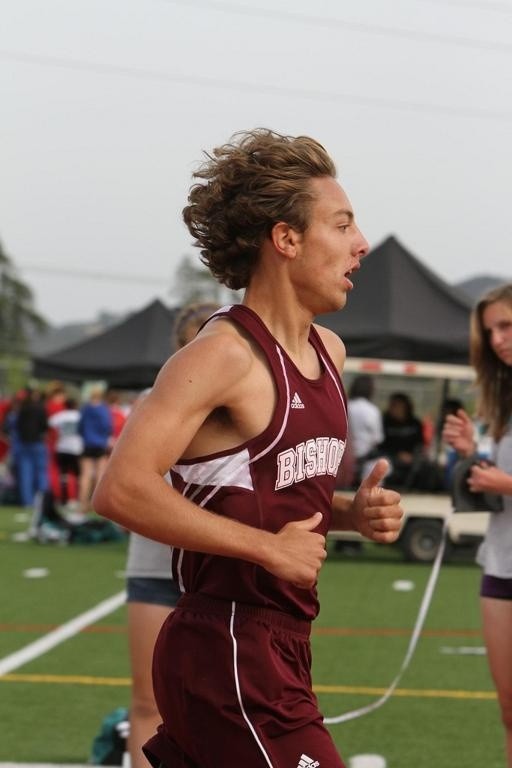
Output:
[1,379,130,515]
[125,305,225,766]
[343,378,466,496]
[441,284,512,768]
[88,129,404,767]
